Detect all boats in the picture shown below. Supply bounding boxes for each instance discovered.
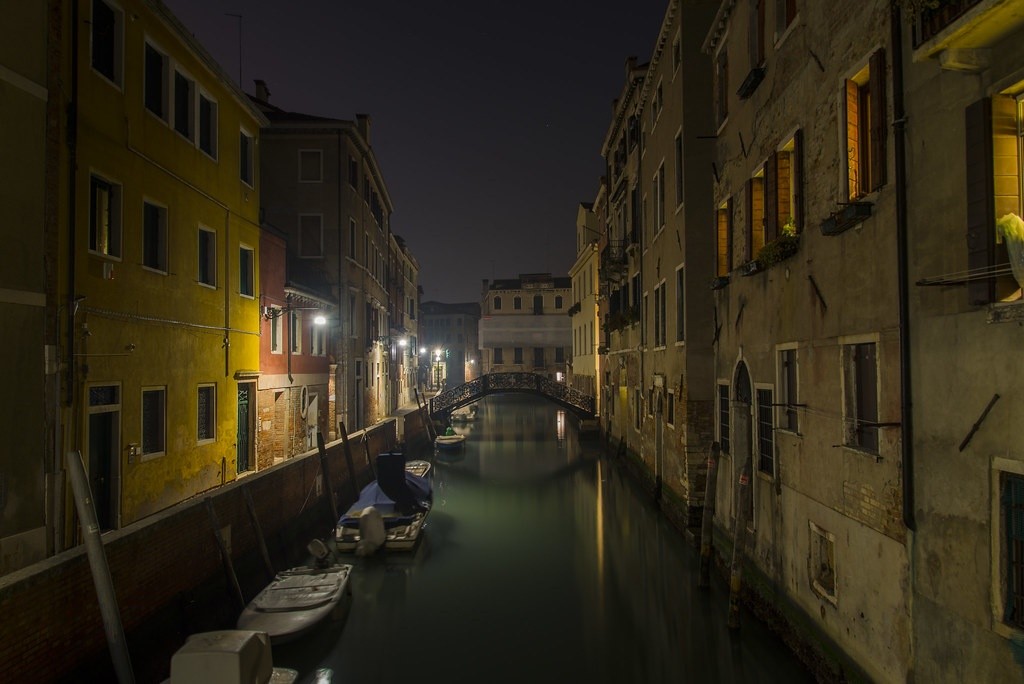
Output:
[234,537,355,649]
[160,629,299,684]
[434,426,469,457]
[334,452,434,557]
